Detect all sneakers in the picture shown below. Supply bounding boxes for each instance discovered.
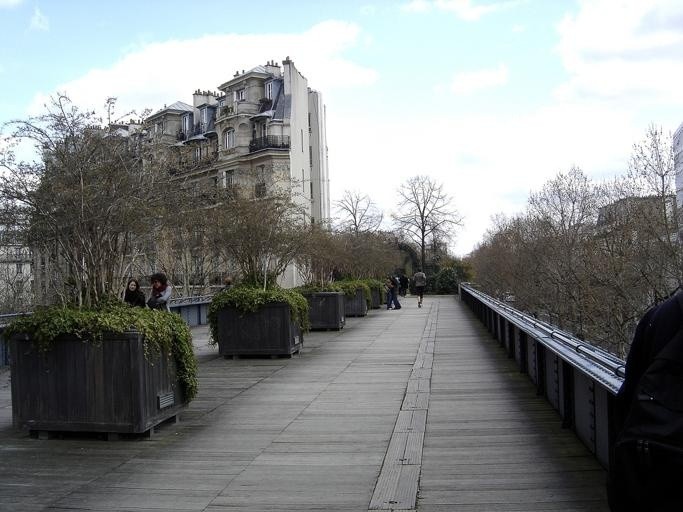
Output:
[387,307,401,309]
[418,302,423,308]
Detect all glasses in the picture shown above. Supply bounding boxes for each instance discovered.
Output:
[152,281,158,284]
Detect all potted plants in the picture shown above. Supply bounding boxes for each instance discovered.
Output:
[334,281,371,317]
[363,279,387,310]
[8,276,200,441]
[205,277,312,359]
[291,279,346,331]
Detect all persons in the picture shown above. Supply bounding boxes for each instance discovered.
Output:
[412,269,426,309]
[221,276,233,292]
[384,271,410,310]
[329,269,337,281]
[147,273,172,314]
[121,279,145,308]
[605,289,681,512]
